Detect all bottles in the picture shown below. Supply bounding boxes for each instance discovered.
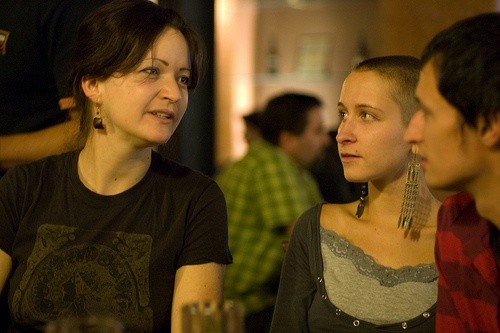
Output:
[179,300,245,333]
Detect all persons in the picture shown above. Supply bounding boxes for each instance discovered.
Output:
[270,55,443,333]
[0,0,500,333]
